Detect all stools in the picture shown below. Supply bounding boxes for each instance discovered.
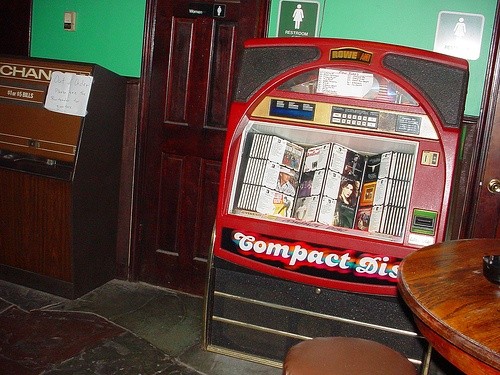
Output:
[283,336,419,375]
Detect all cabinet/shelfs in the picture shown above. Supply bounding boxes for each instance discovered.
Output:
[0,54,126,300]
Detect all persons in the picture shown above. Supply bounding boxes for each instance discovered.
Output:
[282,152,299,169]
[346,153,363,180]
[276,172,296,196]
[338,180,356,208]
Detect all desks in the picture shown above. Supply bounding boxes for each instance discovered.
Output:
[397,239,500,375]
